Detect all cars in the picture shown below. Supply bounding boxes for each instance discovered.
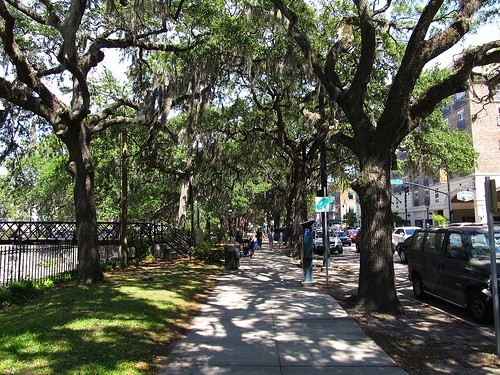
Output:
[396,233,459,265]
[316,224,360,246]
[391,227,422,252]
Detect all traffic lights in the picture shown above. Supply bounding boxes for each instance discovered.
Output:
[405,186,409,193]
[391,154,398,170]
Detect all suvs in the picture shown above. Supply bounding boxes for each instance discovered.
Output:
[311,230,344,255]
[406,225,500,324]
[354,226,362,253]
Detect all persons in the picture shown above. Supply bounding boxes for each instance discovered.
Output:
[268,227,275,250]
[256,228,263,249]
[234,227,243,246]
[249,233,258,256]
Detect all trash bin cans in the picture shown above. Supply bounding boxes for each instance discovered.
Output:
[241,239,252,255]
[223,244,240,270]
